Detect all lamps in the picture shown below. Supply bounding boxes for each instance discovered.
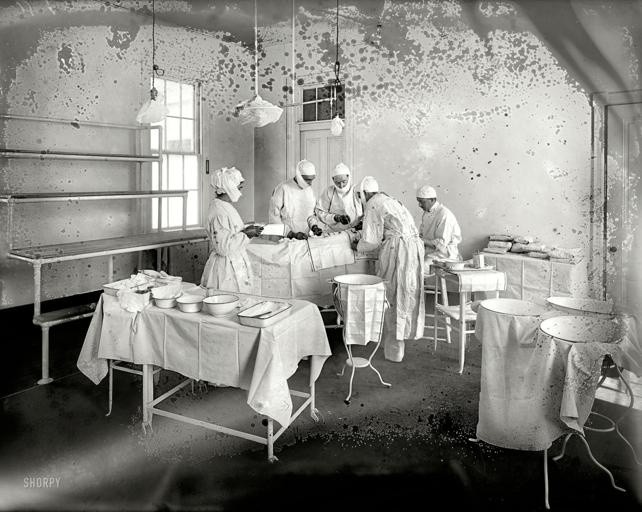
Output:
[136,1,168,125]
[328,1,345,137]
[229,0,284,128]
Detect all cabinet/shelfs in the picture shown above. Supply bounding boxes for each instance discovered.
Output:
[1,109,211,385]
[428,262,508,375]
[99,289,323,467]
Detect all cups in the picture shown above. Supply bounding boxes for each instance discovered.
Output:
[473,254,484,269]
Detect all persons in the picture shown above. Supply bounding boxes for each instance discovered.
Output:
[315,162,362,232]
[200,166,263,388]
[268,160,322,242]
[350,175,426,363]
[416,184,461,277]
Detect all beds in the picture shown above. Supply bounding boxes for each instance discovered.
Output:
[248,234,383,333]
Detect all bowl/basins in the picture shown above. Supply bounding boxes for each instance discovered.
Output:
[446,261,466,271]
[152,292,240,317]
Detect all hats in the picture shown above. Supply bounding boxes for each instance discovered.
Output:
[356,178,378,192]
[210,168,245,195]
[332,165,349,178]
[299,161,315,176]
[416,185,436,198]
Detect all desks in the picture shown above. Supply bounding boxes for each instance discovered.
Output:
[473,248,589,306]
